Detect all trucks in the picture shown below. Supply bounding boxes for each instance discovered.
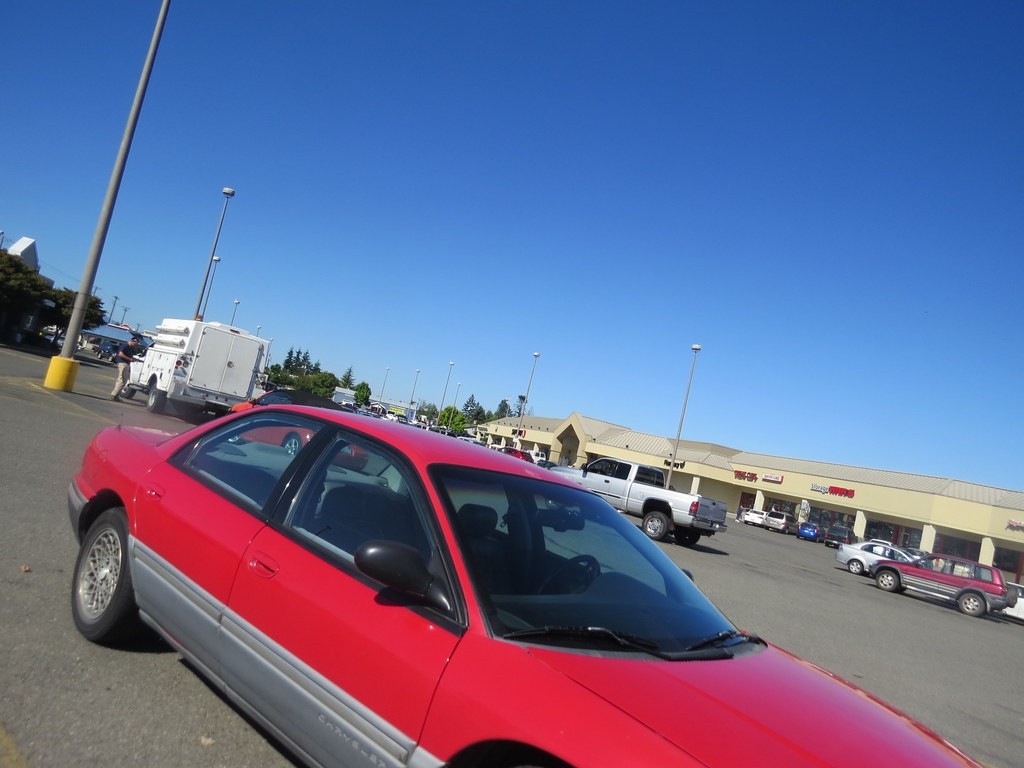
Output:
[118,317,272,417]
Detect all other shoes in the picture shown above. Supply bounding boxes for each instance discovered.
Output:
[109,395,121,402]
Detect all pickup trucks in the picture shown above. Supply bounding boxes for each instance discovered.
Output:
[550,456,728,546]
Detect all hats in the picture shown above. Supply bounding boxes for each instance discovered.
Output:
[132,337,138,342]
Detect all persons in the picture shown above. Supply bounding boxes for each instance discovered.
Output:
[109,337,139,403]
[735,505,744,522]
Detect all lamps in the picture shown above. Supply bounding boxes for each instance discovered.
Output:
[539,427,540,430]
[510,423,512,425]
[524,425,525,427]
[669,453,672,455]
[546,428,548,430]
[504,422,505,425]
[498,421,500,424]
[531,426,532,428]
[593,438,596,442]
[516,424,518,427]
[625,444,629,447]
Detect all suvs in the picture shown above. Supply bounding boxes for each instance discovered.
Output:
[824,524,858,549]
[867,552,1008,617]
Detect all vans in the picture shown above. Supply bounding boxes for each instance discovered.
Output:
[762,510,799,535]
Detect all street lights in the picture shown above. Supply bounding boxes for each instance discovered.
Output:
[191,187,235,319]
[266,338,274,356]
[198,255,222,320]
[230,298,240,326]
[407,369,420,418]
[514,351,540,448]
[447,382,462,436]
[436,361,454,427]
[664,344,702,492]
[378,367,390,404]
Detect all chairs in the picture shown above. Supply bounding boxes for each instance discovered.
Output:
[888,549,895,560]
[457,502,516,595]
[319,485,433,569]
[867,545,873,552]
[942,564,952,574]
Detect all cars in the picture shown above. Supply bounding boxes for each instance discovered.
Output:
[741,508,770,527]
[225,387,367,466]
[867,538,930,561]
[62,403,981,768]
[834,541,916,576]
[796,522,826,543]
[350,388,552,468]
